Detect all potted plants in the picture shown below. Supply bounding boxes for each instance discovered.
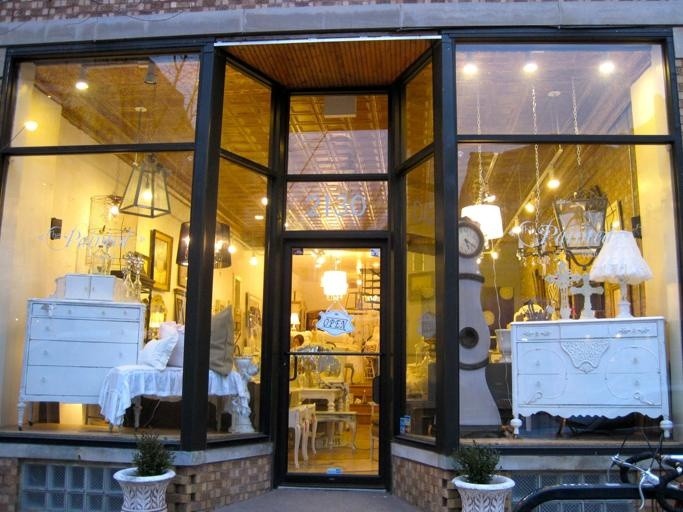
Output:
[450,440,516,512]
[113,436,178,512]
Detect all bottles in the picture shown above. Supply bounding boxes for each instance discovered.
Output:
[92,245,141,303]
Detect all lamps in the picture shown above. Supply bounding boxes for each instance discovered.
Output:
[117,61,172,218]
[550,75,654,318]
[176,220,230,270]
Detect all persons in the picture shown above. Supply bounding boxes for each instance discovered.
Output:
[290,335,304,351]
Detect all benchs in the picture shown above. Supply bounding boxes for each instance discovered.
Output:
[107,363,244,434]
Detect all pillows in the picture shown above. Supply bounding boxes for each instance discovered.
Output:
[141,305,235,378]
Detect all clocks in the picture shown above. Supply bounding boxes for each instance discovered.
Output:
[457,223,484,259]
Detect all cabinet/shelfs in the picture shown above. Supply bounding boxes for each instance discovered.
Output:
[14,298,146,432]
[509,317,674,441]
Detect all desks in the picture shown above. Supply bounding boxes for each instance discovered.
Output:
[288,386,358,469]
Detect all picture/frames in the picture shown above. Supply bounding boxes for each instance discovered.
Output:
[148,228,173,292]
